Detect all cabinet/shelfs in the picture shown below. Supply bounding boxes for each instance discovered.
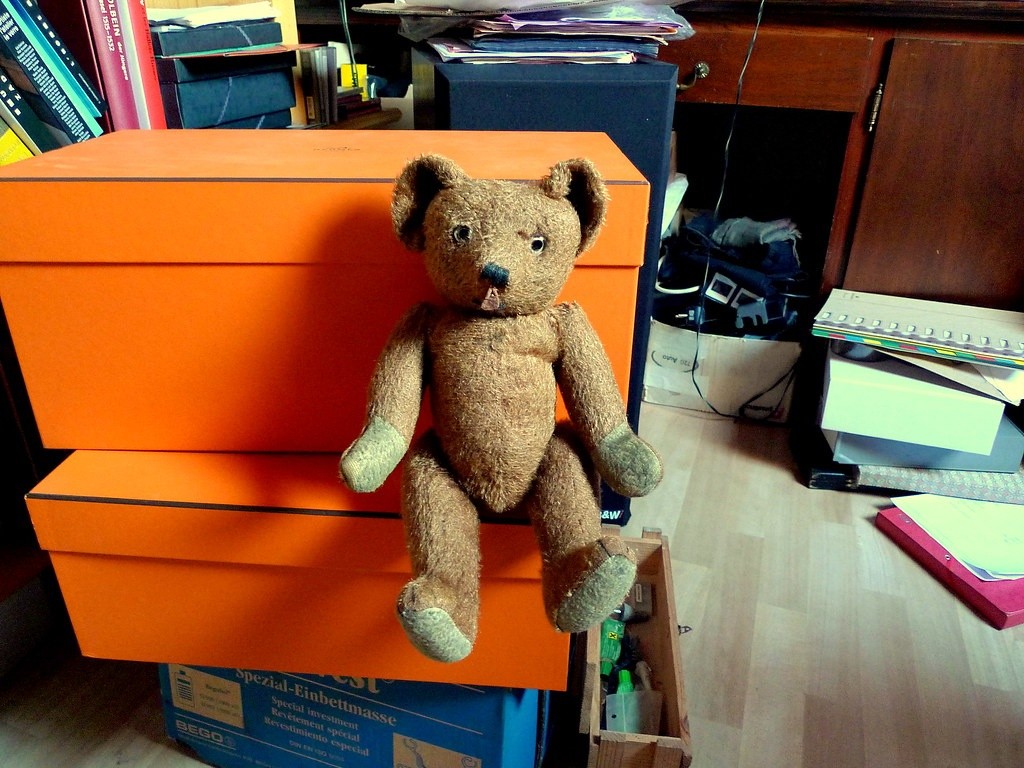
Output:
[656,0,1024,314]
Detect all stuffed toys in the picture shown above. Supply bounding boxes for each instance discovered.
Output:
[336,154,665,663]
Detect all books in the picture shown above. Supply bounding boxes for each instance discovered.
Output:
[426,12,683,77]
[0,0,403,167]
[809,288,1024,632]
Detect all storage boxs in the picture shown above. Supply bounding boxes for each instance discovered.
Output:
[1,23,700,768]
[819,339,1024,507]
